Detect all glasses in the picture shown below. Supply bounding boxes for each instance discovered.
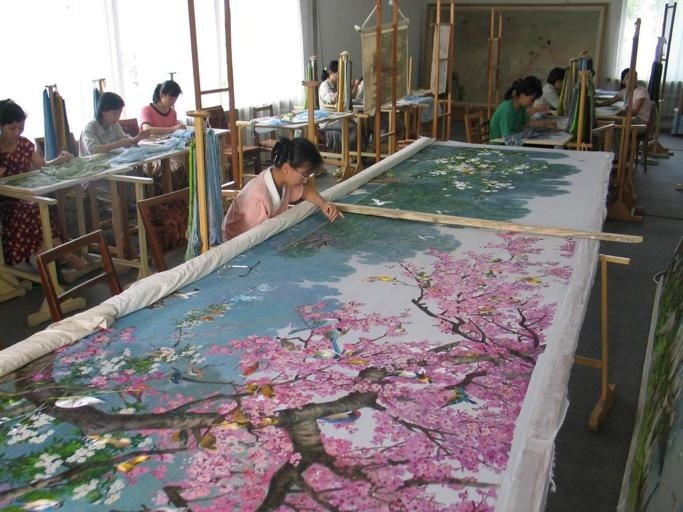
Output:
[286,159,316,180]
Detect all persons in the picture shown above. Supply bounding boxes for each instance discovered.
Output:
[594,68,651,145]
[317,59,367,154]
[77,92,153,224]
[220,136,344,241]
[530,68,565,118]
[490,75,553,147]
[354,77,419,140]
[1,98,88,273]
[138,80,189,198]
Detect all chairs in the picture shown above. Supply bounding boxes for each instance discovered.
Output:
[116,116,140,141]
[627,100,656,172]
[463,111,484,143]
[202,104,262,183]
[248,104,278,175]
[476,117,492,145]
[35,228,122,325]
[135,183,191,273]
[33,131,79,164]
